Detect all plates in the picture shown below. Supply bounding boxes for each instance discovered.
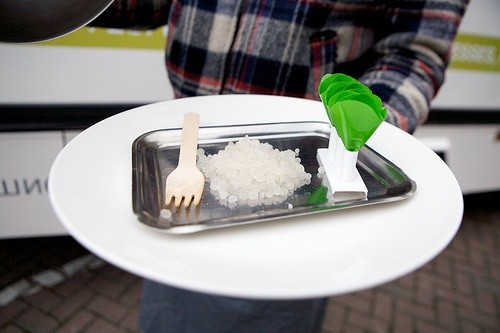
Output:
[48,92,463,300]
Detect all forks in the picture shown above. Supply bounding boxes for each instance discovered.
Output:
[165,112,205,209]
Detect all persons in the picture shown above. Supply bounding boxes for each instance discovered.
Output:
[84,0,470,333]
